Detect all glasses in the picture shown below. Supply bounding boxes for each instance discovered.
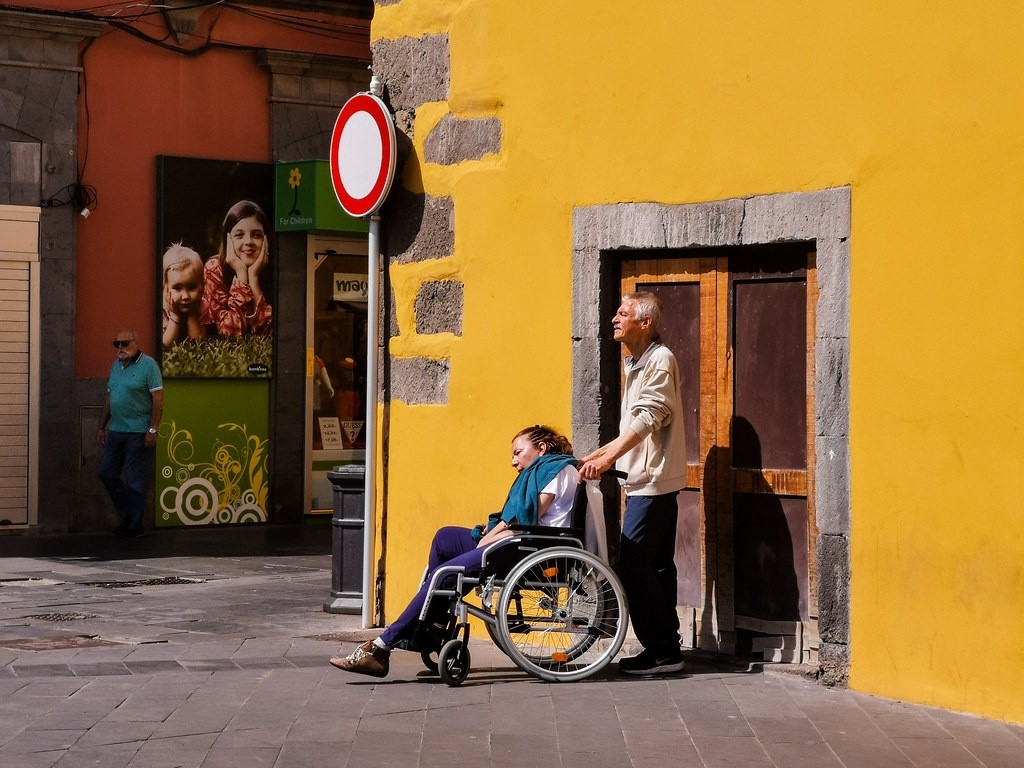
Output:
[110,338,136,348]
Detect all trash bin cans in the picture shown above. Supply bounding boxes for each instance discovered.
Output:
[323,464,365,614]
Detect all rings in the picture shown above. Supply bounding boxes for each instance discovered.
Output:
[264,252,268,256]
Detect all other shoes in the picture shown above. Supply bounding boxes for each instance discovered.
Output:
[111,519,144,536]
[330,640,390,678]
[618,649,685,675]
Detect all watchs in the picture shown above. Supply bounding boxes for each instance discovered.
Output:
[148,428,158,435]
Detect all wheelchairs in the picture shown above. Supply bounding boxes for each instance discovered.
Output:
[418,464,634,688]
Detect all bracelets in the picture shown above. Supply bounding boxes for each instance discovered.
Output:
[168,315,183,325]
[98,425,105,430]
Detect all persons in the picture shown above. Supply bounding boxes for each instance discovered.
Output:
[328,424,583,678]
[313,356,360,419]
[97,329,164,538]
[162,200,273,350]
[577,292,687,674]
